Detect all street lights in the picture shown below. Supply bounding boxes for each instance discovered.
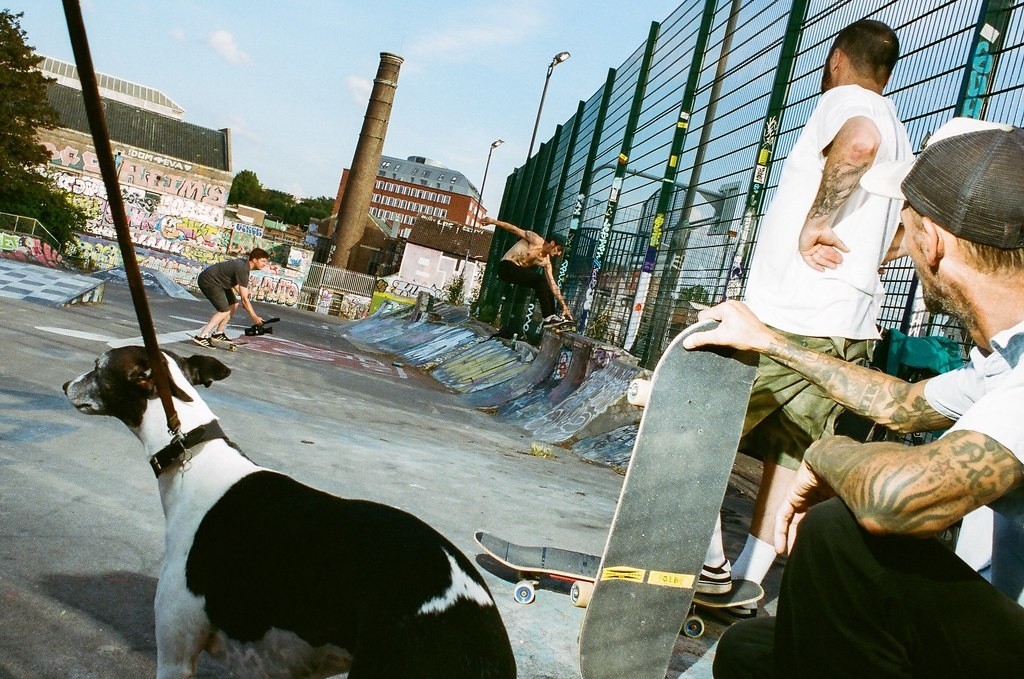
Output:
[459,139,505,280]
[489,51,571,304]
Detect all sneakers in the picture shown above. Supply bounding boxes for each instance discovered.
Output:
[192,335,216,349]
[698,603,758,624]
[211,332,233,343]
[542,316,573,328]
[696,558,732,593]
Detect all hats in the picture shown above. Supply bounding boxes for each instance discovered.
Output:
[900,116,1024,247]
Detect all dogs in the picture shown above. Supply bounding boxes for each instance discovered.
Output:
[58,343,520,679]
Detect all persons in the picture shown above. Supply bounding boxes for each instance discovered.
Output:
[683,117,1024,679]
[479,216,575,328]
[192,248,269,349]
[65,241,116,271]
[696,20,915,617]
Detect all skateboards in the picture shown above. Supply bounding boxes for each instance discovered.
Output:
[577,317,758,679]
[466,526,763,642]
[544,317,576,332]
[186,334,249,351]
